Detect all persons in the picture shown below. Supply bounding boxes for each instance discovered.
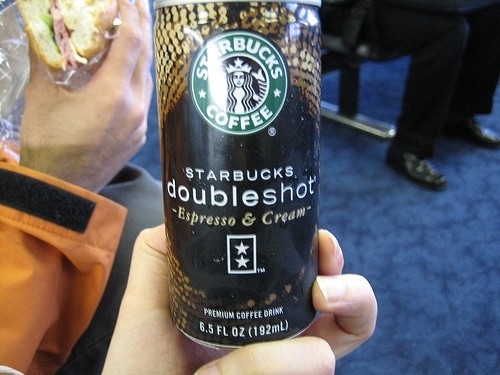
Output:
[0,1,156,375]
[320,0,500,187]
[99,222,384,375]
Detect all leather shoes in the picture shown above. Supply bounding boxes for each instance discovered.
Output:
[446,115,500,147]
[385,145,447,189]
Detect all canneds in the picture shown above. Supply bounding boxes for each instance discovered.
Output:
[152,0,323,354]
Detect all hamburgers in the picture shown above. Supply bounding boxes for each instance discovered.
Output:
[15,0,120,69]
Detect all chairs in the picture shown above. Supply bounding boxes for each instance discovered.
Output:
[318,32,407,139]
